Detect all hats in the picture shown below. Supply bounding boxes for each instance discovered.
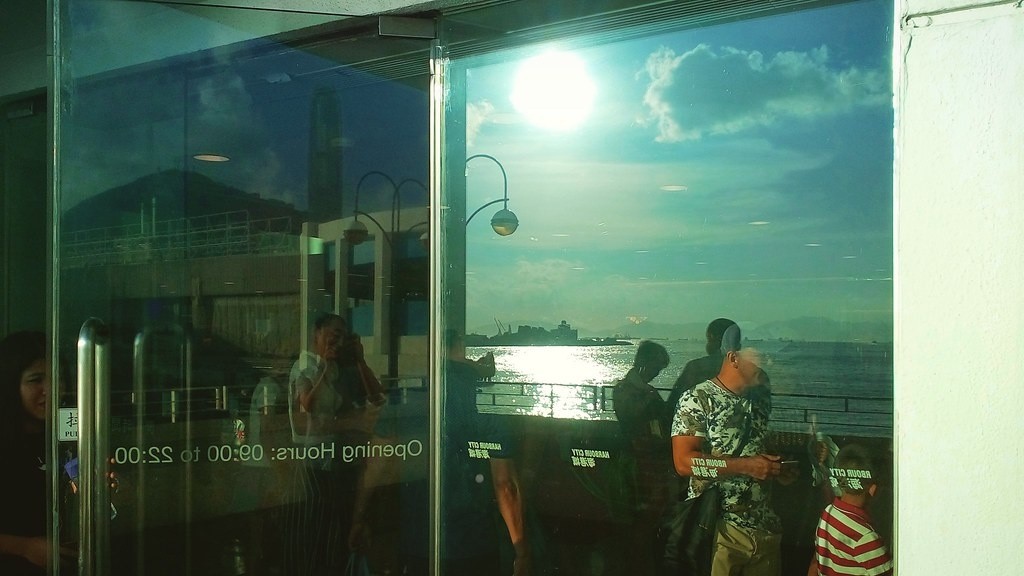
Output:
[720,319,790,356]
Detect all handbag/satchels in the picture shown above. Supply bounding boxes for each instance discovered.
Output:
[655,474,720,576]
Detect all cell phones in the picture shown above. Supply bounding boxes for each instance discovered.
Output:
[780,459,800,466]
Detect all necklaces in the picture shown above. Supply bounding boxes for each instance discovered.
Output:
[715,376,739,396]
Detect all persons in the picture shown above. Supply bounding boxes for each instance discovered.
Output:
[0,313,893,576]
